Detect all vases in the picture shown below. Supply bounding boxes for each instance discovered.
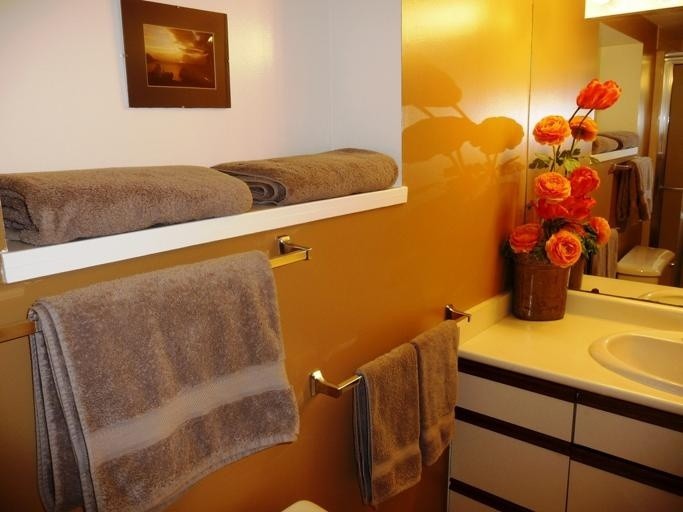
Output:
[569,254,588,290]
[512,252,571,321]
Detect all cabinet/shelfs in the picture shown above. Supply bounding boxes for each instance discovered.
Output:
[446,356,683,512]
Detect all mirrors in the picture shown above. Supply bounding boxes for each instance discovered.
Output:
[525,0,683,309]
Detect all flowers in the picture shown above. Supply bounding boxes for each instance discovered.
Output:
[501,78,608,268]
[530,80,622,257]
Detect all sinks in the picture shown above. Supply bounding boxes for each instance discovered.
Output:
[638,289,683,307]
[588,330,683,398]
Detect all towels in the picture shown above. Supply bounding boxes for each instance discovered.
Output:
[592,129,653,279]
[354,320,459,507]
[26,249,300,512]
[0,166,253,247]
[211,148,399,207]
